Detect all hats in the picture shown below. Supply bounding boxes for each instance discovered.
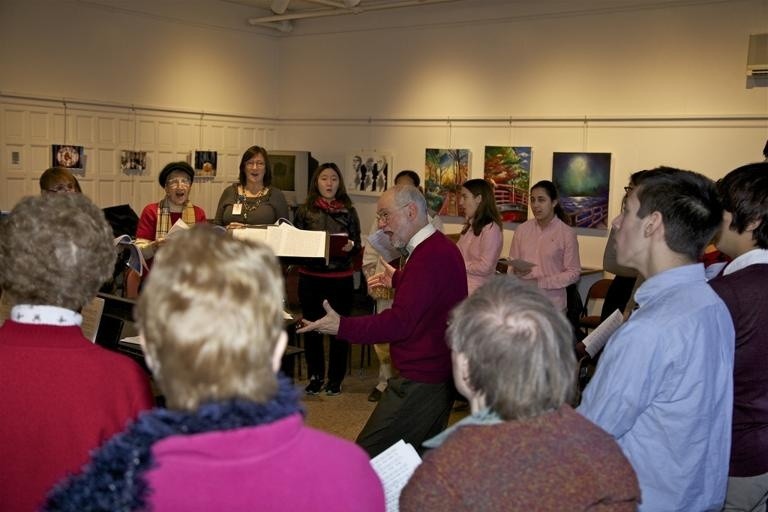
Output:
[158,161,195,188]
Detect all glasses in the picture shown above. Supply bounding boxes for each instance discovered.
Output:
[376,203,409,222]
[624,186,633,191]
[244,160,265,169]
[47,189,78,194]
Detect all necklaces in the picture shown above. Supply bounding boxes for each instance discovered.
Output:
[238,181,270,216]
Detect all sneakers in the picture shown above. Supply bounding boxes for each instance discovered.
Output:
[368,388,382,402]
[325,382,342,395]
[304,375,324,394]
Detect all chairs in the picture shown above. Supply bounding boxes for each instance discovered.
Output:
[579,279,610,335]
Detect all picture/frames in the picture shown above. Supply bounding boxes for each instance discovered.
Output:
[424,147,470,218]
[119,151,148,172]
[484,146,528,225]
[551,150,610,239]
[49,143,85,172]
[342,154,394,196]
[192,151,219,179]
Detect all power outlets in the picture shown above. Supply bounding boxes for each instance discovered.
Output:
[11,151,19,164]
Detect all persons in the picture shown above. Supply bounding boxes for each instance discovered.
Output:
[372,156,388,192]
[43,227,385,512]
[293,162,363,398]
[215,144,288,229]
[709,163,768,512]
[295,184,469,455]
[504,178,580,317]
[352,155,367,191]
[134,161,206,238]
[599,170,653,318]
[398,274,642,512]
[370,168,420,263]
[33,166,82,197]
[453,179,503,296]
[361,156,376,191]
[0,193,154,435]
[574,166,735,511]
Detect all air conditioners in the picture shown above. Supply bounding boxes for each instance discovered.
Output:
[745,33,768,78]
